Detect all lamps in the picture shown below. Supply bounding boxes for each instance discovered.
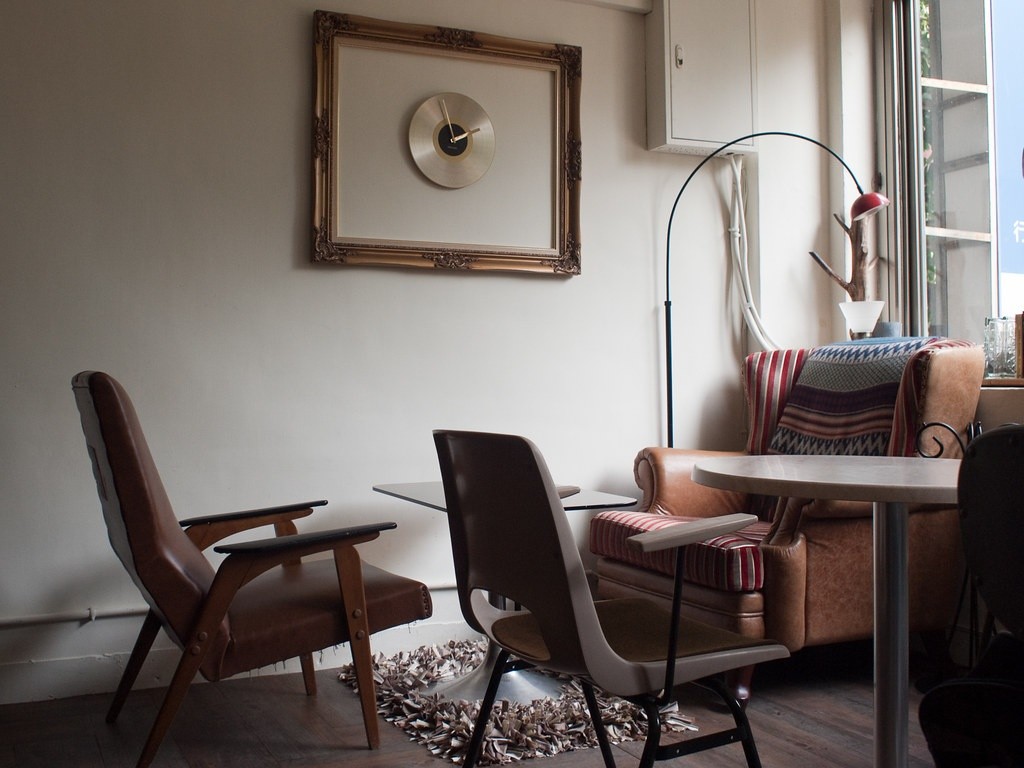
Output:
[664,132,891,446]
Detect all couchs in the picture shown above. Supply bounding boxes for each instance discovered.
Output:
[589,335,989,705]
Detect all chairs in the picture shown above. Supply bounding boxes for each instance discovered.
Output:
[433,429,789,768]
[916,423,1024,768]
[68,371,434,754]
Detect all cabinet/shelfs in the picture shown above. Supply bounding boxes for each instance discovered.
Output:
[909,0,993,366]
[642,0,761,158]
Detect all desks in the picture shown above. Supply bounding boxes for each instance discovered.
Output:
[370,474,638,707]
[692,452,956,768]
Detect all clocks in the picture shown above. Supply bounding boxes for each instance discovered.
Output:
[304,9,582,277]
